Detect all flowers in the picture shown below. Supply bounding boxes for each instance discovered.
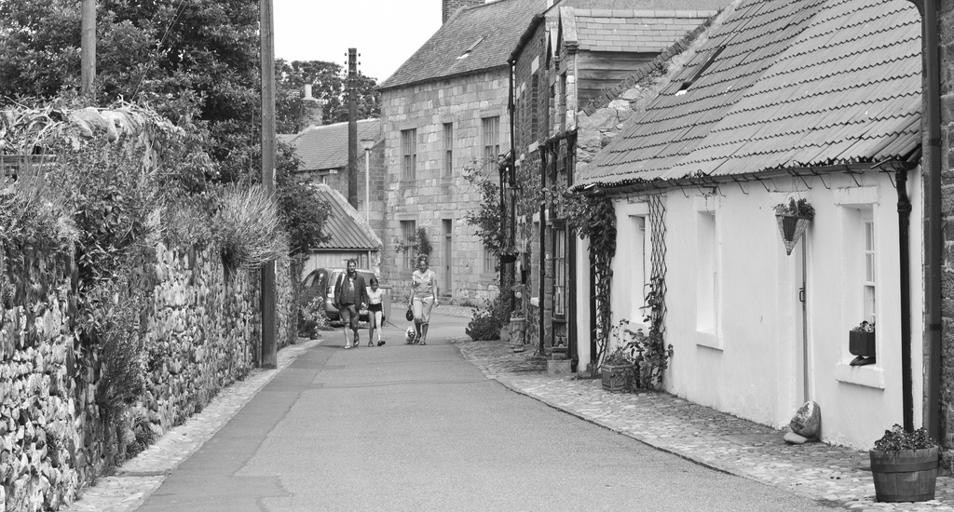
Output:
[775,199,816,217]
[853,321,875,333]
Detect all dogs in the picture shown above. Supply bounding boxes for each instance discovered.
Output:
[405,326,416,344]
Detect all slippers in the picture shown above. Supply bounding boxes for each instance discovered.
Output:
[377,341,385,346]
[344,344,351,349]
[353,334,359,347]
[368,342,373,347]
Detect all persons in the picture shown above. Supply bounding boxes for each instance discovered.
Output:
[409,254,440,345]
[334,259,367,349]
[361,277,385,347]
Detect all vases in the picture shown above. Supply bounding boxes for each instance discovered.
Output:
[779,217,810,254]
[849,330,875,366]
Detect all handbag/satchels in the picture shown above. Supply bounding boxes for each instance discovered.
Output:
[406,305,413,321]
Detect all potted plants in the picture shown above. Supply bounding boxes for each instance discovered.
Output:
[600,320,642,393]
[871,427,939,501]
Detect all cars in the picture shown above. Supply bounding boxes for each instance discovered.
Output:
[299,267,384,328]
